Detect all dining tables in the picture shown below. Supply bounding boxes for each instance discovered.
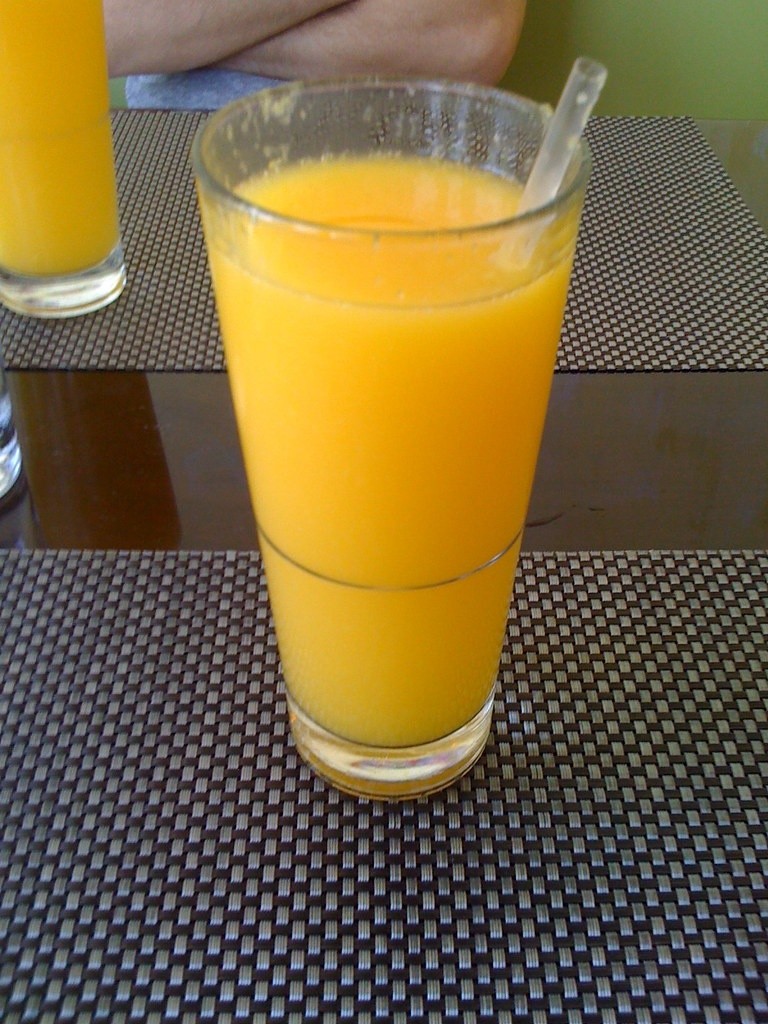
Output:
[0,105,767,1024]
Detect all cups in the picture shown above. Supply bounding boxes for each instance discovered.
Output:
[0,1,127,318]
[187,73,595,802]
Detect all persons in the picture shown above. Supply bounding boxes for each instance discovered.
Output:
[99,0,536,111]
